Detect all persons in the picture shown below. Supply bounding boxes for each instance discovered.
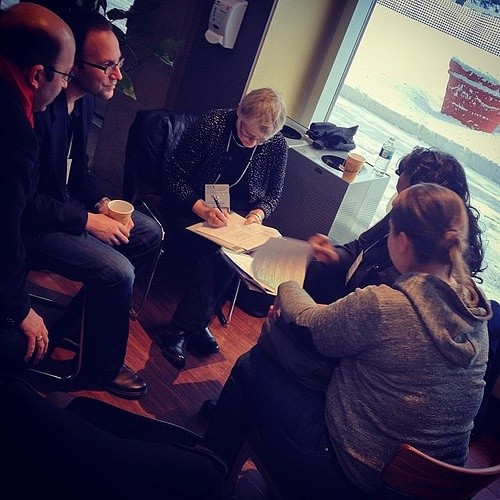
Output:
[202,181,493,500]
[37,12,162,398]
[305,147,485,304]
[156,89,289,369]
[0,2,76,369]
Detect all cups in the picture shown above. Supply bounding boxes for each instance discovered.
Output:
[107,199,134,229]
[341,153,366,183]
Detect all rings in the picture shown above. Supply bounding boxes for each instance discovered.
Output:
[37,335,43,341]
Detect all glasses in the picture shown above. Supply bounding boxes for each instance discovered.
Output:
[84,56,126,74]
[240,121,273,144]
[53,68,74,83]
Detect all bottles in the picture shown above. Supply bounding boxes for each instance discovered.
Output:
[370,136,397,177]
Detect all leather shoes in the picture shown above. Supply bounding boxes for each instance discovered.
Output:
[155,327,187,364]
[107,364,147,397]
[173,320,218,355]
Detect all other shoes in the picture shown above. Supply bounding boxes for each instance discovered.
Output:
[202,399,218,417]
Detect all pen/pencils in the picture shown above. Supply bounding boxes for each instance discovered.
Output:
[213,196,222,212]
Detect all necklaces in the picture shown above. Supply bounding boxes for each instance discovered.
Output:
[213,131,257,188]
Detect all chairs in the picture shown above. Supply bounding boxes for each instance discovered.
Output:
[375,442,500,500]
[22,262,91,384]
[123,109,236,330]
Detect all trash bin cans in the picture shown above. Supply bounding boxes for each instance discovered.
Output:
[260,143,391,242]
[280,115,314,148]
